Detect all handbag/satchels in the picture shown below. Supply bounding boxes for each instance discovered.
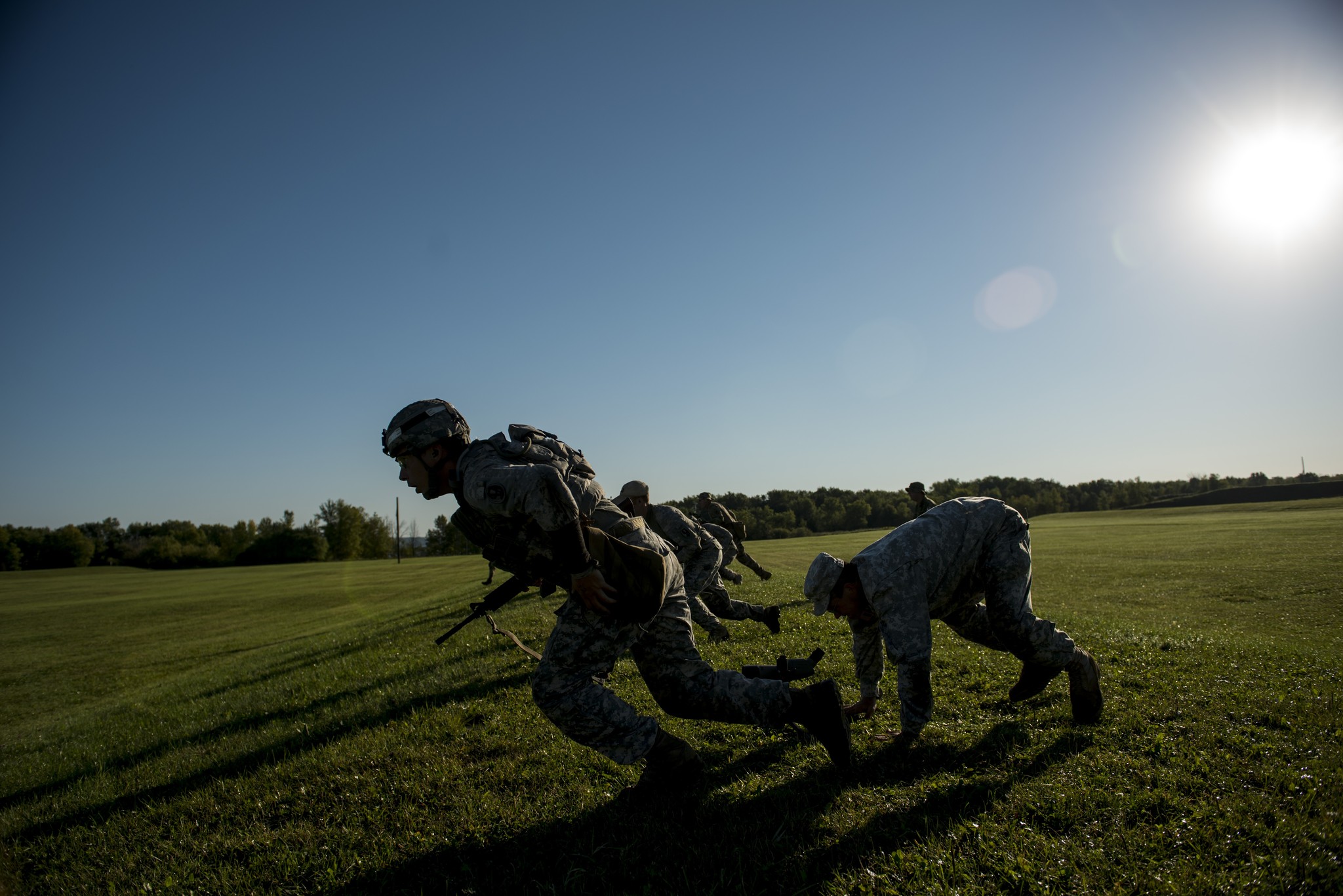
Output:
[732,521,746,538]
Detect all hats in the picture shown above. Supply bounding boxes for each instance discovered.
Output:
[804,552,844,616]
[382,398,470,458]
[688,515,702,523]
[905,482,928,495]
[611,481,649,505]
[697,492,715,501]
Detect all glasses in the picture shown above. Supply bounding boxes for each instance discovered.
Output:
[828,591,843,613]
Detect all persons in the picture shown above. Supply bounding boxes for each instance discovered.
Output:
[805,495,1105,758]
[700,521,744,588]
[382,397,857,816]
[904,482,934,518]
[697,492,773,582]
[618,480,782,644]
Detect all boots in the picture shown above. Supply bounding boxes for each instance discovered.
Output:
[707,625,730,645]
[1065,644,1104,726]
[619,722,704,802]
[788,677,851,770]
[733,572,743,585]
[1009,658,1063,702]
[761,569,772,581]
[762,605,781,635]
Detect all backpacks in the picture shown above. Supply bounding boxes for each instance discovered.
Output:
[570,527,666,623]
[488,424,596,482]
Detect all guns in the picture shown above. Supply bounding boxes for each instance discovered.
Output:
[435,574,533,646]
